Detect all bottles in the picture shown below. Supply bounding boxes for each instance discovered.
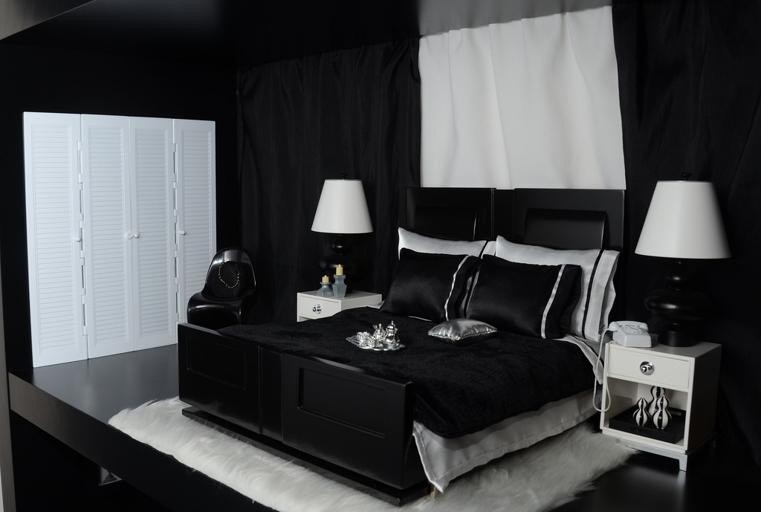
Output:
[374,325,396,348]
[319,275,346,299]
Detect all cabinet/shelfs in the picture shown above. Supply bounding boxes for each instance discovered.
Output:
[173,119,217,324]
[79,114,178,360]
[23,111,88,369]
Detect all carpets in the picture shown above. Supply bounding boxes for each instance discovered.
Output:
[109,396,638,512]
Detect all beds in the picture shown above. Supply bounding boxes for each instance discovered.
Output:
[178,189,633,503]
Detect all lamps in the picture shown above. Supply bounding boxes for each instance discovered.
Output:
[311,179,373,292]
[634,179,731,346]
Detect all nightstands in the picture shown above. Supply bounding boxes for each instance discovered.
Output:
[599,341,725,473]
[297,290,383,324]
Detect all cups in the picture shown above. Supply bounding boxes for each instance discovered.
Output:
[357,331,375,347]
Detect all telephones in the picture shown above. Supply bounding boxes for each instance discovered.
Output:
[608,321,652,348]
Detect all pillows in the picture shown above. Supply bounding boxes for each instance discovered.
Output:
[379,226,621,339]
